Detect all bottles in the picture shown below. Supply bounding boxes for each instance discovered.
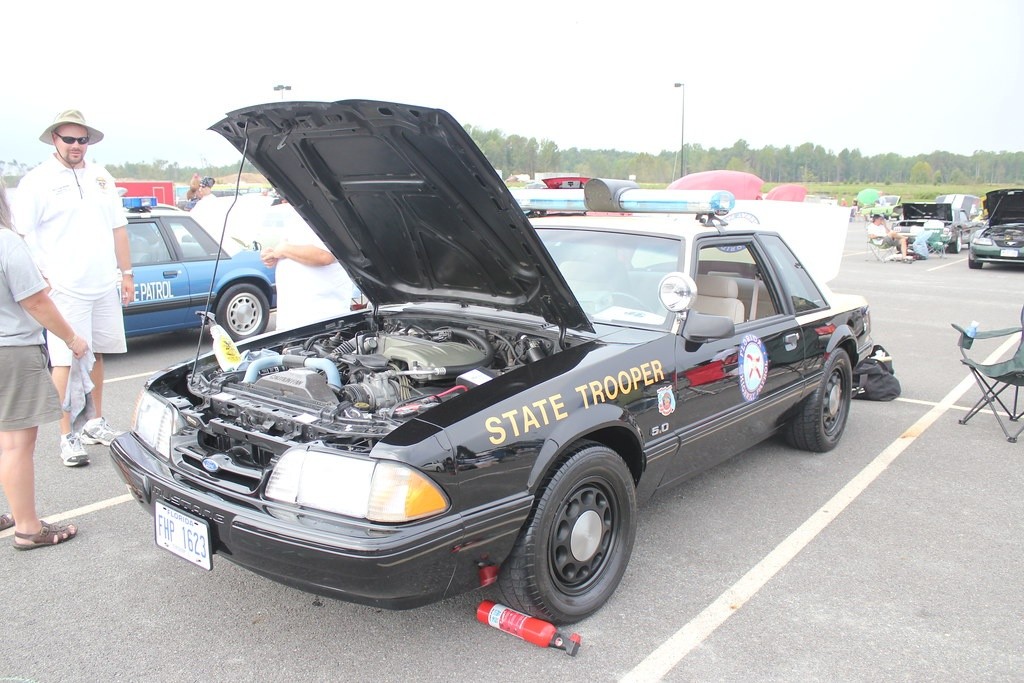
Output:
[966,321,979,338]
[475,601,581,656]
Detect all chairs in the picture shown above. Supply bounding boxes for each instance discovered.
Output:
[951,305,1024,443]
[694,274,745,326]
[130,238,153,263]
[867,219,950,263]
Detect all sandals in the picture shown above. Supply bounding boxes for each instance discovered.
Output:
[0,513,15,530]
[13,520,78,551]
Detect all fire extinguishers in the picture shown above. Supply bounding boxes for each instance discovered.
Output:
[474,599,583,656]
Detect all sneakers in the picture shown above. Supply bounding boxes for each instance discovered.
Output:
[61,433,90,466]
[80,416,127,446]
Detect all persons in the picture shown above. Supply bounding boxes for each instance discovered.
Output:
[851,199,858,216]
[868,214,912,264]
[262,198,352,329]
[875,191,885,207]
[841,198,846,206]
[0,175,88,551]
[184,177,216,212]
[9,108,134,413]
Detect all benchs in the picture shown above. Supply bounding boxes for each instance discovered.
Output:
[625,269,776,322]
[154,241,207,261]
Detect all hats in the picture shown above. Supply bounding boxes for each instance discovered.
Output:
[39,109,104,145]
[200,176,215,188]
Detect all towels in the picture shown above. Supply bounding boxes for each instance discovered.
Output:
[226,347,280,372]
[61,344,96,438]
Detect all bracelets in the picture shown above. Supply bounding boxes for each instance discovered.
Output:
[122,269,134,277]
[67,334,77,349]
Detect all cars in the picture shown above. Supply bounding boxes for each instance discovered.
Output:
[118,187,276,342]
[879,188,1024,269]
[110,99,873,625]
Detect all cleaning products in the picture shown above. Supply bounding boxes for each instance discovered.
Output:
[195,311,241,372]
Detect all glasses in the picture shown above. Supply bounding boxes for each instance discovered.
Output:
[199,184,206,188]
[54,131,89,145]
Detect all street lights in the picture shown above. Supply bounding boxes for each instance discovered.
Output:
[674,83,685,178]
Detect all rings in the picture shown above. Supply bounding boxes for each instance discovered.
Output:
[85,352,87,354]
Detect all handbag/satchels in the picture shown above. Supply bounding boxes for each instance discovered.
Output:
[851,345,901,402]
